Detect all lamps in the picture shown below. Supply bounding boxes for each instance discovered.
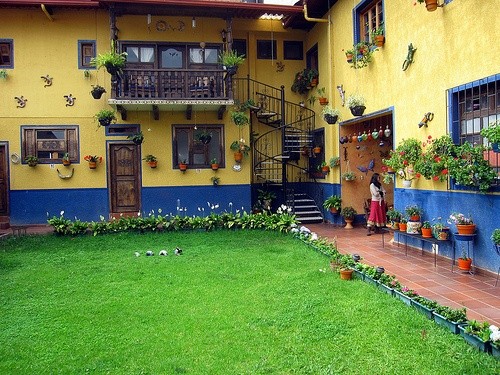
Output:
[115,26,119,39]
[220,28,228,43]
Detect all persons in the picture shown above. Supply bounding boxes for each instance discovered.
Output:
[367,173,388,236]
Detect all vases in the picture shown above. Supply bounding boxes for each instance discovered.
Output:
[421,227,433,238]
[388,167,395,173]
[403,180,412,189]
[381,285,416,306]
[490,342,500,357]
[326,115,338,124]
[425,0,438,11]
[455,223,476,234]
[432,227,449,241]
[410,215,420,222]
[351,106,365,116]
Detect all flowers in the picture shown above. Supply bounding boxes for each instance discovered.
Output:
[489,325,500,346]
[46,199,317,245]
[321,105,341,122]
[414,0,424,6]
[345,93,364,110]
[406,203,473,237]
[380,273,418,297]
[382,138,448,182]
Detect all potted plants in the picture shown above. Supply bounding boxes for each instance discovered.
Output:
[398,217,408,232]
[385,209,402,230]
[322,196,343,214]
[311,236,379,288]
[25,47,250,186]
[434,121,500,192]
[458,249,472,271]
[257,189,276,207]
[291,69,327,105]
[342,207,357,229]
[411,294,491,352]
[342,170,358,183]
[342,24,386,70]
